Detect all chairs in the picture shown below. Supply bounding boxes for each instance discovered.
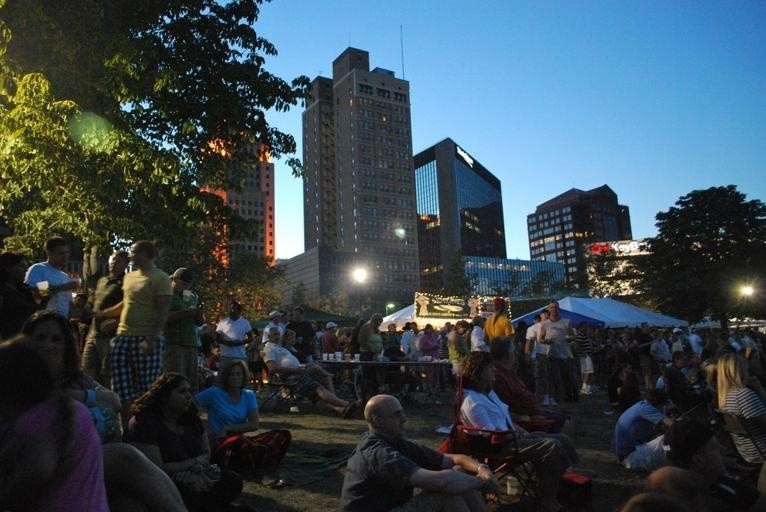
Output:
[661,377,712,423]
[704,410,757,485]
[448,422,549,512]
[255,358,313,415]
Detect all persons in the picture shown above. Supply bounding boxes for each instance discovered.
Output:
[110,243,198,406]
[128,360,292,512]
[570,320,765,394]
[341,395,501,511]
[614,354,765,512]
[197,300,261,387]
[456,336,578,496]
[0,238,185,510]
[0,235,199,298]
[260,299,571,418]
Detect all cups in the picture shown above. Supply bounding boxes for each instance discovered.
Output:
[323,352,360,361]
[506,475,518,495]
[36,281,49,297]
[427,355,432,362]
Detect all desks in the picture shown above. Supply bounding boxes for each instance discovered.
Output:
[318,359,459,408]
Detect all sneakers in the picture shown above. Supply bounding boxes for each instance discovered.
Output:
[580,388,592,394]
[341,398,363,417]
[543,398,560,407]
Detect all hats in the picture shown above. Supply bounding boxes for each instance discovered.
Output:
[326,322,336,328]
[268,310,284,319]
[672,327,684,334]
[168,266,195,284]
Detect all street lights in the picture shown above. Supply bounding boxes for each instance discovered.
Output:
[393,226,406,307]
[352,267,366,317]
